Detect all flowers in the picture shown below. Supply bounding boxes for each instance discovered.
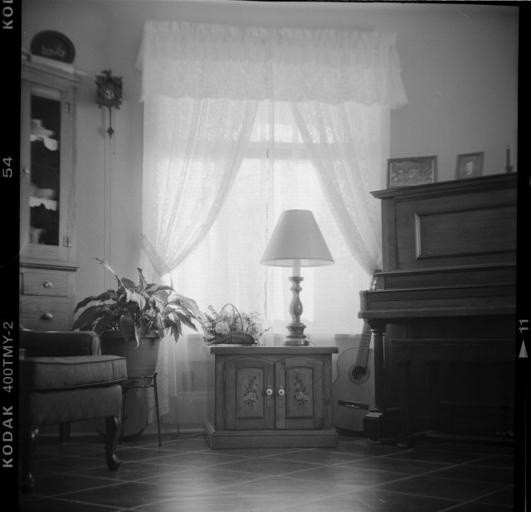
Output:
[193,301,272,350]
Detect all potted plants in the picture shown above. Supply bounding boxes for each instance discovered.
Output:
[67,249,209,390]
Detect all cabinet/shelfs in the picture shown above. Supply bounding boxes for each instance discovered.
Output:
[14,258,81,336]
[198,337,342,452]
[19,52,94,271]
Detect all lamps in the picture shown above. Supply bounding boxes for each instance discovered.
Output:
[259,205,337,347]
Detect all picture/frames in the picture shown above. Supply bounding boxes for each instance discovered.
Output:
[456,150,485,181]
[387,154,439,190]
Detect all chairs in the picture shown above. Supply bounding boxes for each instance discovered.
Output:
[17,323,129,494]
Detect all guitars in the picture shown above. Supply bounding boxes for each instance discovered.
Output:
[332,269,385,430]
